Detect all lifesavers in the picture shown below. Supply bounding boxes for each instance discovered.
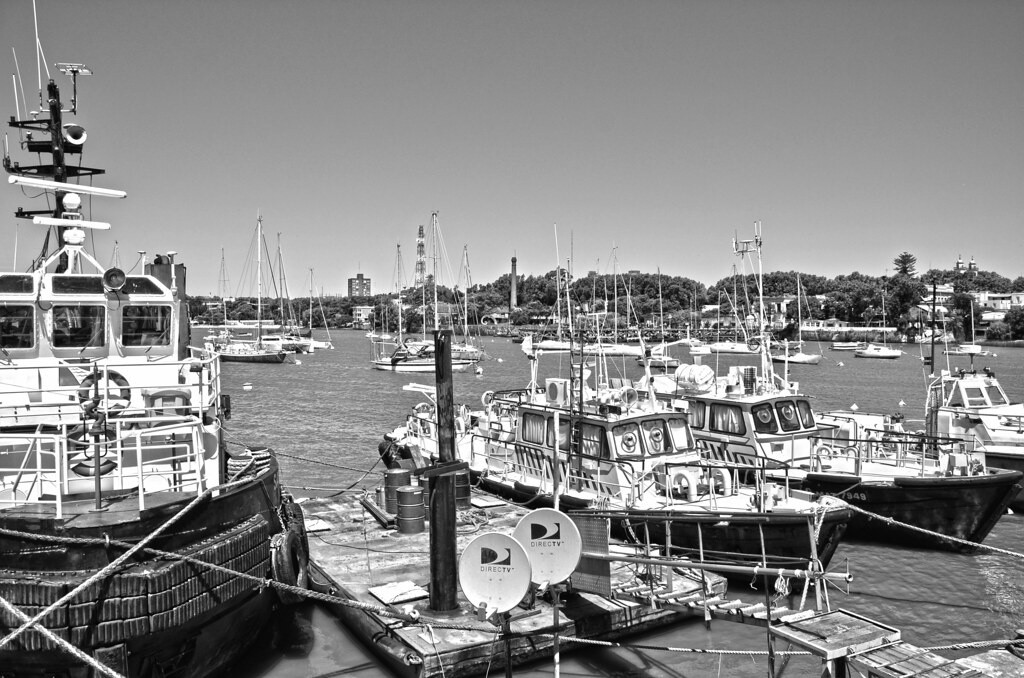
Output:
[413,402,434,421]
[62,423,124,477]
[481,390,498,408]
[377,440,403,470]
[817,446,834,461]
[79,369,131,419]
[747,337,760,351]
[270,484,311,609]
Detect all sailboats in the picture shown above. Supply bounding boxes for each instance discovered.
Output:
[0,0,1024,677]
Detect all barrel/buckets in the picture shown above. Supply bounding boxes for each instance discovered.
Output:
[396,485,425,534]
[456,470,471,509]
[419,478,430,521]
[384,468,411,514]
